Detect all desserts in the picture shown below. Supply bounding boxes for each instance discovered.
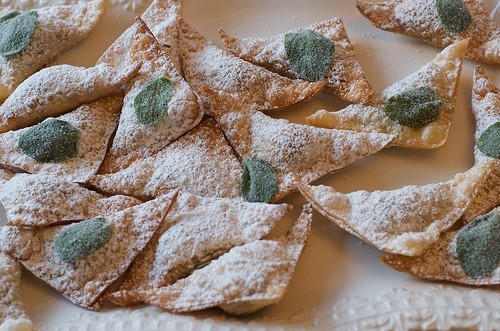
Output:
[0,1,500,331]
